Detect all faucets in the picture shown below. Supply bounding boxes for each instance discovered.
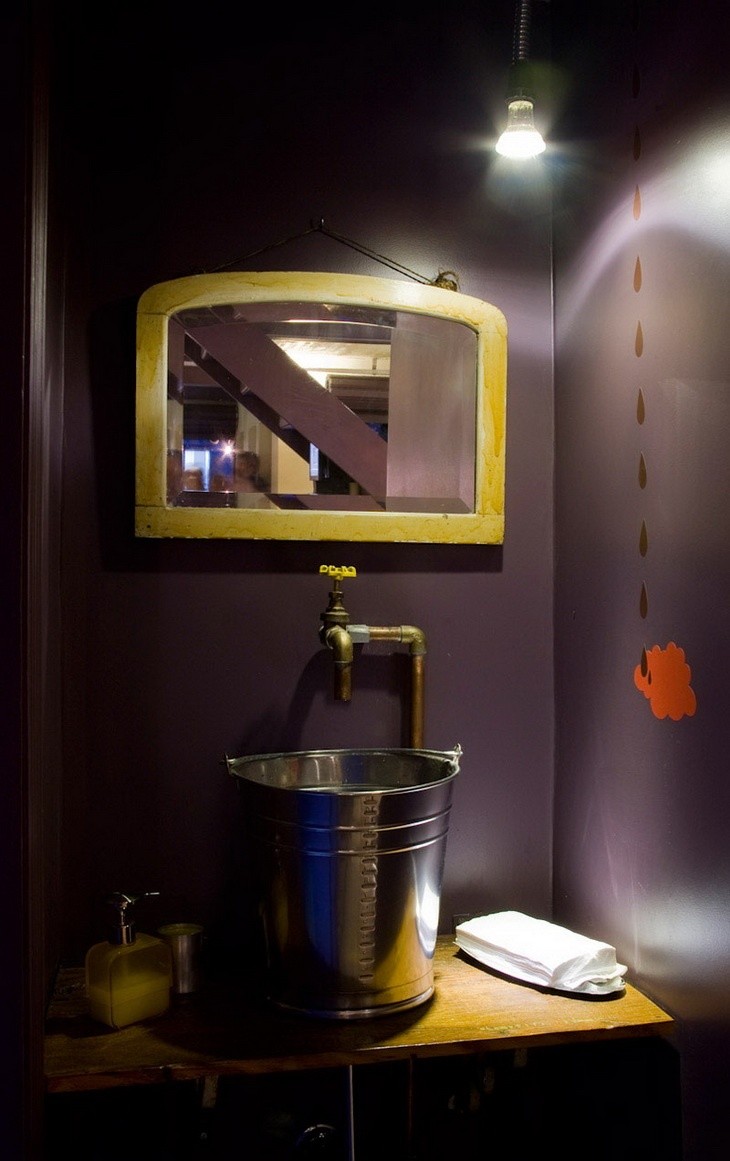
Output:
[314,561,359,703]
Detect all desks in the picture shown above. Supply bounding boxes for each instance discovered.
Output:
[41,917,687,1104]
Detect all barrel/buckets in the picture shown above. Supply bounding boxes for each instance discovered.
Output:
[220,743,464,1019]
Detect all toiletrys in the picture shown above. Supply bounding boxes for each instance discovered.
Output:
[85,888,174,1029]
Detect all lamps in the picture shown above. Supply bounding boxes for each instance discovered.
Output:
[495,0,546,161]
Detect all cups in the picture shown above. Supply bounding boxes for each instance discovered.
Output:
[157,923,207,996]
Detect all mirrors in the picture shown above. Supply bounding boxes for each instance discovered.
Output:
[125,267,512,549]
